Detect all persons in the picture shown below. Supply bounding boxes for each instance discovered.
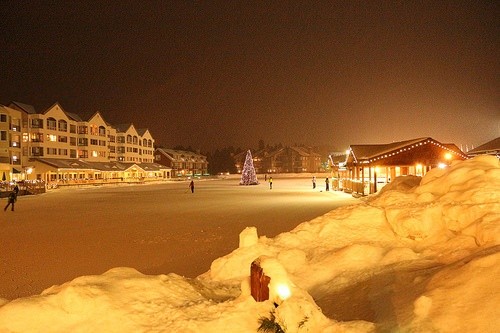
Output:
[269,177,272,190]
[325,178,329,191]
[189,181,194,193]
[312,177,316,189]
[4,186,19,211]
